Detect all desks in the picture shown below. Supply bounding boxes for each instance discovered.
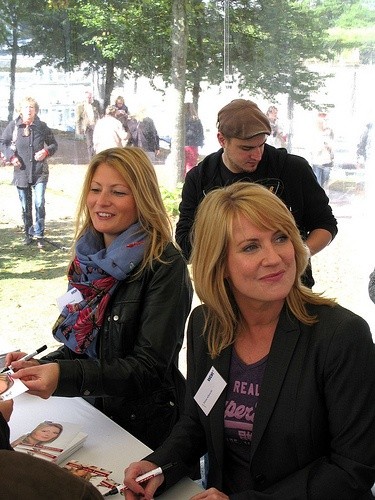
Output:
[323,168,363,203]
[0,349,225,500]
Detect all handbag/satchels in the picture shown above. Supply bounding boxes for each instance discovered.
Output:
[3,121,18,166]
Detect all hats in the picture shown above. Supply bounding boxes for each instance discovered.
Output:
[218,99,272,140]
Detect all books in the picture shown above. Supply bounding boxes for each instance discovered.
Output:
[14,425,88,465]
[64,460,121,495]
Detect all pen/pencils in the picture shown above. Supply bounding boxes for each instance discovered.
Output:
[0,345,49,375]
[102,464,174,498]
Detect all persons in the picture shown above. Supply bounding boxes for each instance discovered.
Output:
[175,100,338,287]
[0,451,105,500]
[6,149,193,448]
[182,103,204,176]
[2,97,57,248]
[0,397,14,450]
[0,372,14,394]
[124,180,375,500]
[22,421,62,446]
[264,105,374,186]
[74,89,160,161]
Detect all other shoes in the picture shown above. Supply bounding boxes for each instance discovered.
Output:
[24,237,32,245]
[37,239,45,248]
